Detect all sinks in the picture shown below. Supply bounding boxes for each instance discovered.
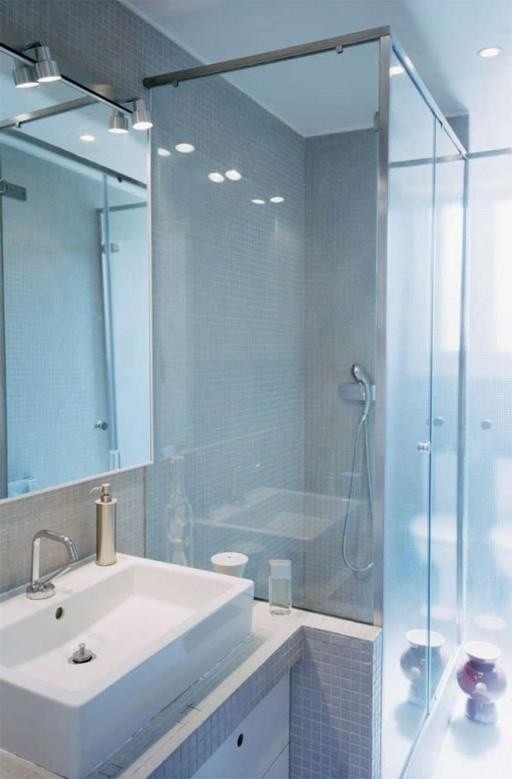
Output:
[194,483,362,597]
[0,548,254,779]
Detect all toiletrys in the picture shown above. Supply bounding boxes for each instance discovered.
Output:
[265,556,294,616]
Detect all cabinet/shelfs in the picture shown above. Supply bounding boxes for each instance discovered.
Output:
[191,669,290,778]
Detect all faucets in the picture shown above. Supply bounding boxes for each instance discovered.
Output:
[24,528,79,599]
[226,461,263,506]
[25,481,35,493]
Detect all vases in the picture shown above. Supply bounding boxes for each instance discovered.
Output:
[398,630,450,708]
[456,638,509,724]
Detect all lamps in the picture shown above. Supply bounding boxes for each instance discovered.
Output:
[107,108,130,135]
[129,93,152,131]
[13,62,39,89]
[33,41,62,82]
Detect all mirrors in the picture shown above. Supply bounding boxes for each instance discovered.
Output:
[0,46,157,501]
